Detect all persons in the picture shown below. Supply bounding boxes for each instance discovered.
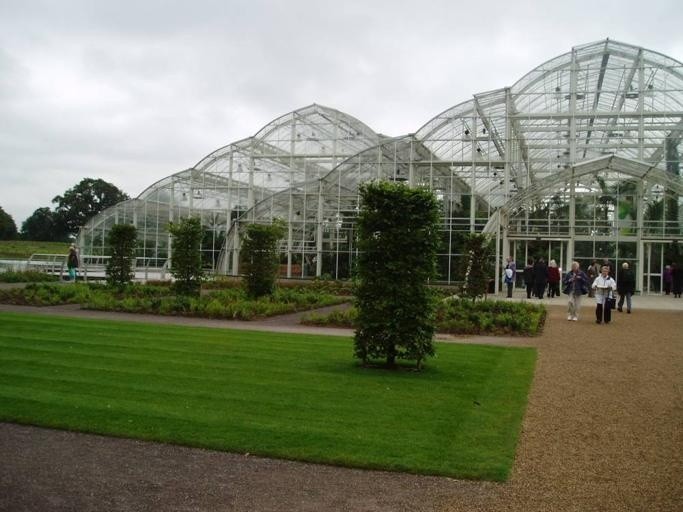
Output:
[591,266,616,324]
[663,264,672,295]
[672,262,683,298]
[67,246,78,283]
[504,257,614,299]
[616,263,635,313]
[561,261,590,320]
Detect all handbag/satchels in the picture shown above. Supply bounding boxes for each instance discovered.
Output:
[605,288,616,309]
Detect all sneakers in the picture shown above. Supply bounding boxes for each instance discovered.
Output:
[595,319,609,324]
[567,315,578,322]
[617,307,623,313]
[506,293,560,299]
[627,309,631,314]
[665,293,681,298]
